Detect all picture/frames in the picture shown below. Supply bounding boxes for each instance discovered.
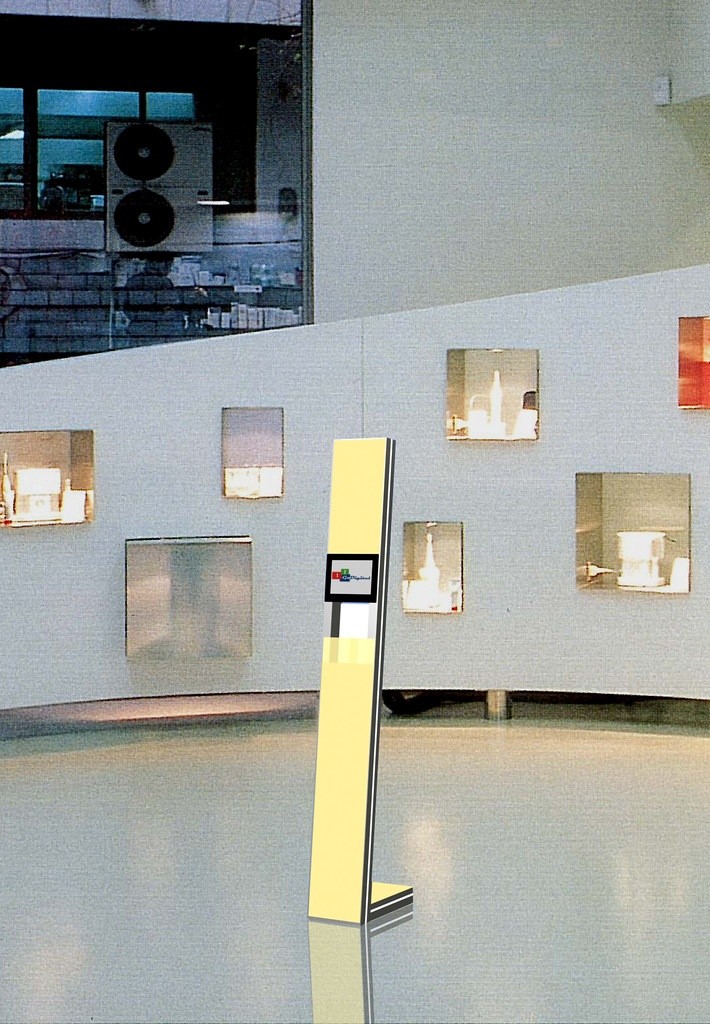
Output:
[324,554,377,603]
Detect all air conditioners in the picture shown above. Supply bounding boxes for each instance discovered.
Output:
[105,120,213,252]
